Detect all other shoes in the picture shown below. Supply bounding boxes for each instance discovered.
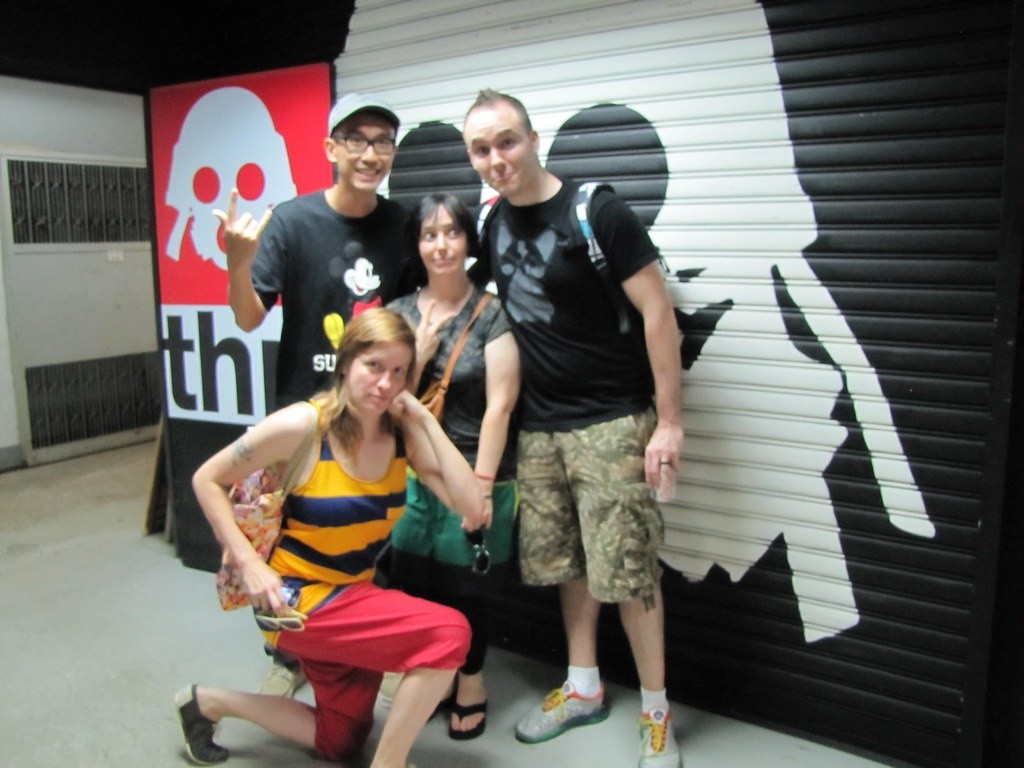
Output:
[257,663,307,698]
[377,670,404,703]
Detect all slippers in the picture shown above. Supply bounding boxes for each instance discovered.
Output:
[448,697,487,740]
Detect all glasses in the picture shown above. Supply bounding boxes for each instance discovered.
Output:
[465,530,490,573]
[335,134,396,157]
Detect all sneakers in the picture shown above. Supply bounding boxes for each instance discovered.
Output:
[513,678,610,742]
[640,710,681,768]
[178,685,231,765]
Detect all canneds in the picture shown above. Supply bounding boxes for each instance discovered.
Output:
[649,461,677,502]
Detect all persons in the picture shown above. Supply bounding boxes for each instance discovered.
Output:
[213,92,429,413]
[462,90,685,768]
[379,192,521,740]
[173,306,486,768]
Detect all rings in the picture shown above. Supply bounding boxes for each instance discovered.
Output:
[660,460,669,465]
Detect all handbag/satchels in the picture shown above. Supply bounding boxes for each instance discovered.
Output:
[419,389,447,425]
[215,400,324,612]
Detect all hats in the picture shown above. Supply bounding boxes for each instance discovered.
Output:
[326,93,401,138]
